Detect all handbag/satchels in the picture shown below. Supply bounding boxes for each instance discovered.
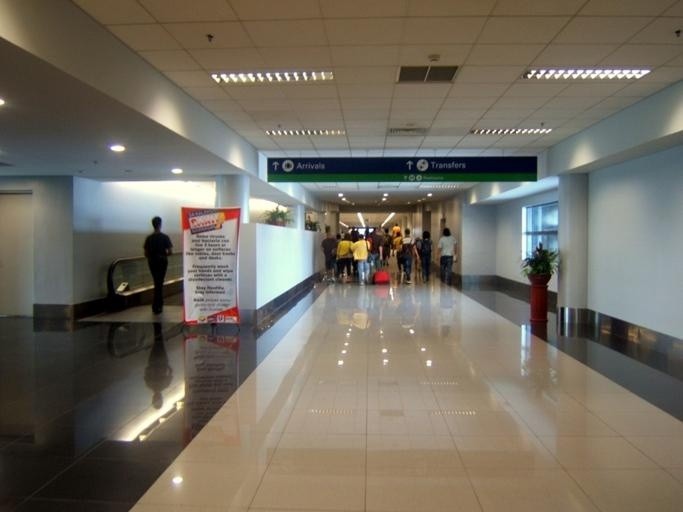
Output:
[368,253,372,263]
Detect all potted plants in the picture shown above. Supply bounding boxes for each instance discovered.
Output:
[518,242,559,284]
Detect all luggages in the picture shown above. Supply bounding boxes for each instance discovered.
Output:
[374,271,390,284]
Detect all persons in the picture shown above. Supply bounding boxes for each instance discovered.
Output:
[144,217,172,313]
[319,223,457,286]
